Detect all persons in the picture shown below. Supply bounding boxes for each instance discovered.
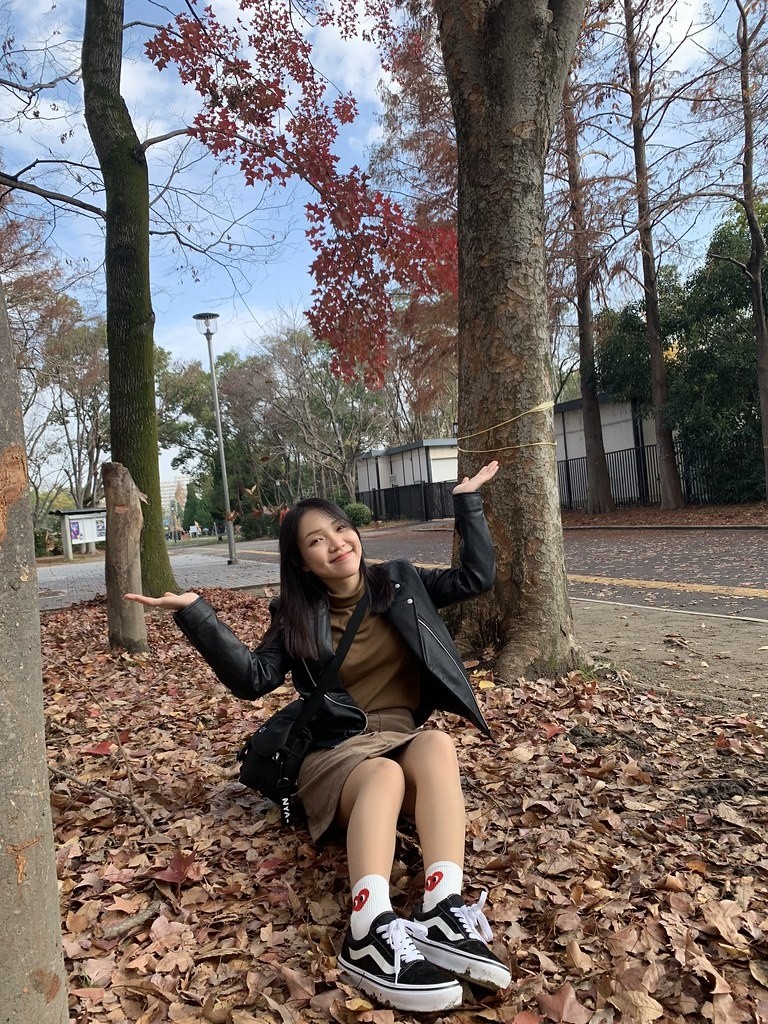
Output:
[280,504,291,525]
[124,460,510,1012]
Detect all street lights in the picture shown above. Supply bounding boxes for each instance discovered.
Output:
[193,312,238,565]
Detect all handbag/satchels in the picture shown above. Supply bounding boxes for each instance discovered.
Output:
[238,697,319,803]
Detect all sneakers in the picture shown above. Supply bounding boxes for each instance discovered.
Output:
[404,891,511,992]
[336,910,463,1012]
